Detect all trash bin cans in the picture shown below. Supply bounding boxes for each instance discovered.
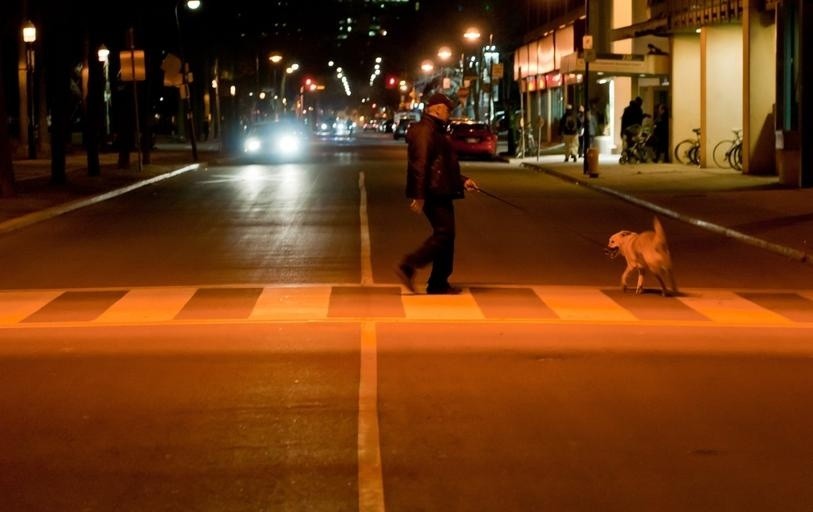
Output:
[586,148,600,178]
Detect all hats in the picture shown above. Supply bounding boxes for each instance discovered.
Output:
[428,93,456,108]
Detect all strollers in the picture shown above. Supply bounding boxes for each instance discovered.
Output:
[620,125,656,164]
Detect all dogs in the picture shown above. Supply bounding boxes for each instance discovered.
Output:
[608,216,678,297]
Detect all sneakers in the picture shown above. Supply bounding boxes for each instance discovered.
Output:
[395,266,415,293]
[426,286,462,293]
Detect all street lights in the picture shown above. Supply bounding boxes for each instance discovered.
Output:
[23,21,37,157]
[582,32,593,174]
[97,45,114,151]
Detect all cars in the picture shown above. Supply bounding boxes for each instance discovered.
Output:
[315,117,353,137]
[446,116,497,161]
[242,119,308,163]
[363,119,418,143]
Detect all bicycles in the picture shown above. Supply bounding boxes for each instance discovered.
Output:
[675,128,701,165]
[712,129,744,171]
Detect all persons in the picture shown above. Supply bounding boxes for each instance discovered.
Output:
[621,97,653,159]
[647,105,669,162]
[560,101,597,163]
[394,95,478,293]
